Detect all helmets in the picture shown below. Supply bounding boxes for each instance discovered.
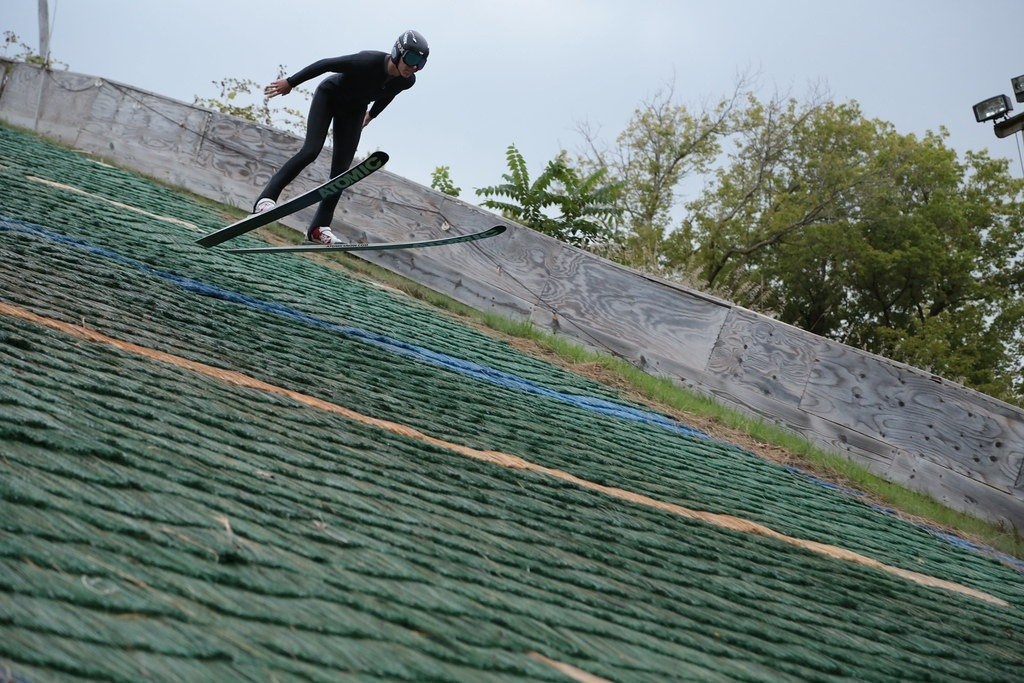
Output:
[391,30,430,65]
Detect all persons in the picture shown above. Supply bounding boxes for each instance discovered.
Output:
[253,29,429,245]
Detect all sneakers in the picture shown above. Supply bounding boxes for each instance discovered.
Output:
[305,226,341,245]
[254,198,276,213]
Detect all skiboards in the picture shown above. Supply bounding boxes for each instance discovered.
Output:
[194,149,508,256]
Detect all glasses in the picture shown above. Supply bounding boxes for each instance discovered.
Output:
[401,51,427,70]
